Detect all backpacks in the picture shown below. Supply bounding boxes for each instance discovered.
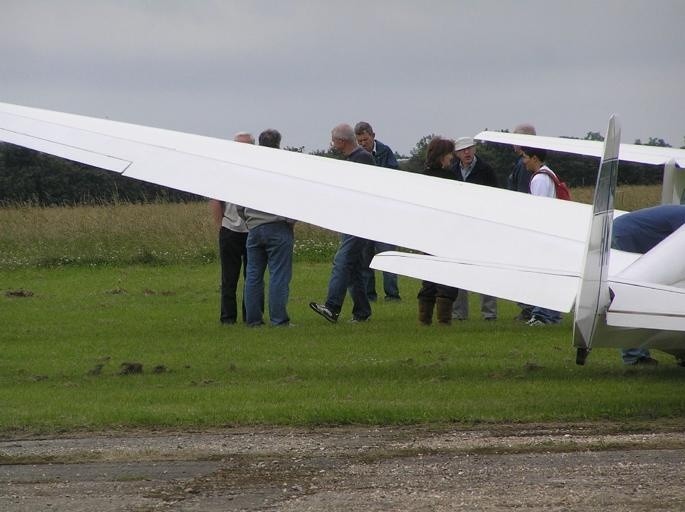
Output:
[528,169,572,201]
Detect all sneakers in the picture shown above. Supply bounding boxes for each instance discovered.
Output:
[513,310,546,328]
[310,302,371,329]
[632,356,659,365]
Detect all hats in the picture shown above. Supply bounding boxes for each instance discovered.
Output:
[453,137,474,152]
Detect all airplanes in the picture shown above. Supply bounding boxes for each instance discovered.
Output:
[0,101,684,366]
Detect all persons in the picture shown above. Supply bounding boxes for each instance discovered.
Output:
[309,123,376,321]
[610,205,685,365]
[211,134,256,323]
[418,138,459,325]
[235,130,297,327]
[354,122,402,301]
[450,136,497,320]
[509,124,536,319]
[520,146,560,326]
